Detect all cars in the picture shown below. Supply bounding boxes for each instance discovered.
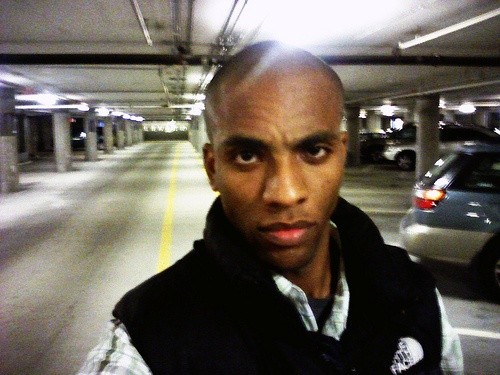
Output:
[398,143,500,304]
[383,123,500,170]
[71,127,117,151]
[359,133,388,161]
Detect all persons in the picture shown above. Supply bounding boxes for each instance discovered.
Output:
[77,38,467,375]
